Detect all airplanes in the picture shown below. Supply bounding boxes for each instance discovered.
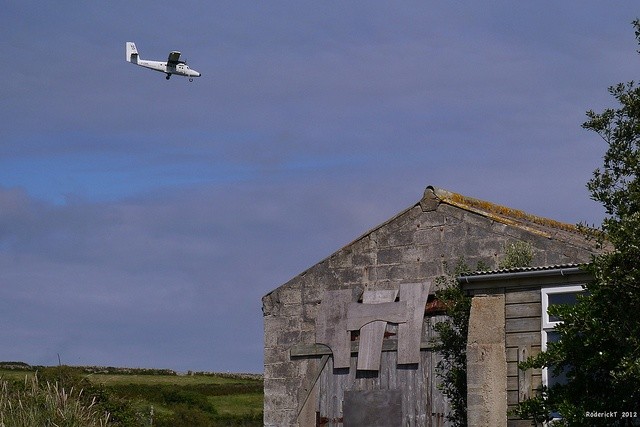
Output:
[126,41,201,82]
